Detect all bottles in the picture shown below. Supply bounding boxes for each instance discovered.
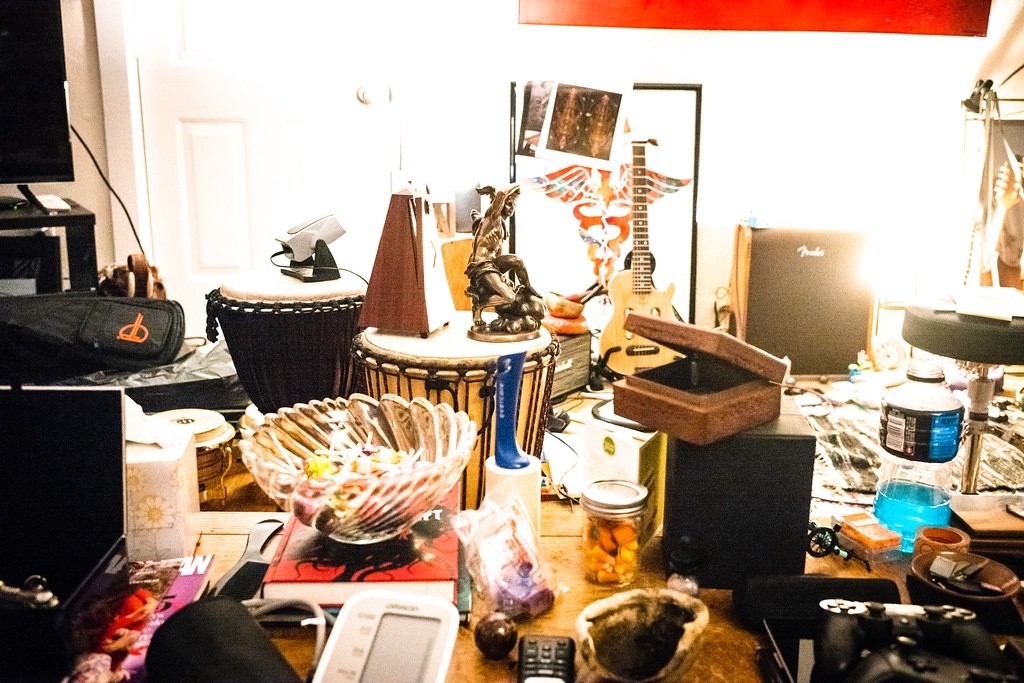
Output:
[873,366,965,556]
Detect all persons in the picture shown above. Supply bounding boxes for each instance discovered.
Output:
[463,184,546,331]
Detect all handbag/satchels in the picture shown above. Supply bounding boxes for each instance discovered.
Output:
[730,222,881,377]
[0,293,186,382]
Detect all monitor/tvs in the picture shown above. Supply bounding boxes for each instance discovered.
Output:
[0,0,76,215]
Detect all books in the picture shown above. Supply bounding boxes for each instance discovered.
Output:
[61,553,217,683]
[262,480,471,626]
[950,494,1024,556]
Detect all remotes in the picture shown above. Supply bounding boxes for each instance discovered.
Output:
[517,635,576,683]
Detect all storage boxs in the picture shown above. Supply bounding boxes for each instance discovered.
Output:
[0,384,129,683]
[730,225,878,373]
[662,396,817,590]
[612,314,787,445]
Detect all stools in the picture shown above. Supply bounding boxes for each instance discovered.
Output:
[901,307,1024,495]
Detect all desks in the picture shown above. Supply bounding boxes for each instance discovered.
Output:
[0,199,99,299]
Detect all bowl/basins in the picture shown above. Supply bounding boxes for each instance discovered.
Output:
[239,393,477,545]
[911,551,1022,600]
